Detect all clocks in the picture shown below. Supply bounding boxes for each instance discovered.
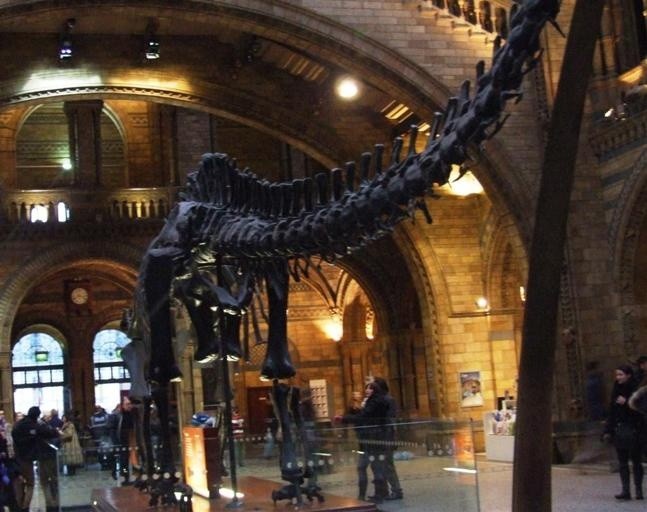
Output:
[69,286,89,306]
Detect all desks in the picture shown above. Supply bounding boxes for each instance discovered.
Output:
[480,411,515,464]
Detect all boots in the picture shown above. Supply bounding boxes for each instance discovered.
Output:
[368,478,385,503]
[633,465,644,499]
[614,463,630,500]
[358,481,367,501]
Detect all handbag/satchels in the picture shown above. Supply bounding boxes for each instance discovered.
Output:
[611,420,639,450]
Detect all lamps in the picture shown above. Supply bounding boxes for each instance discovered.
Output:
[145,19,161,61]
[57,18,75,64]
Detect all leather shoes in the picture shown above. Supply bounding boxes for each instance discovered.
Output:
[368,493,403,501]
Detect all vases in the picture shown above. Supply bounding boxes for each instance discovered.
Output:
[550,431,579,464]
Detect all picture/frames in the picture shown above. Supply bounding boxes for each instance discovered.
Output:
[457,369,485,409]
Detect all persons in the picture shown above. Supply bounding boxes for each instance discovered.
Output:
[604,354,647,499]
[370,376,404,500]
[262,395,280,460]
[334,378,389,503]
[229,407,247,469]
[0,389,161,511]
